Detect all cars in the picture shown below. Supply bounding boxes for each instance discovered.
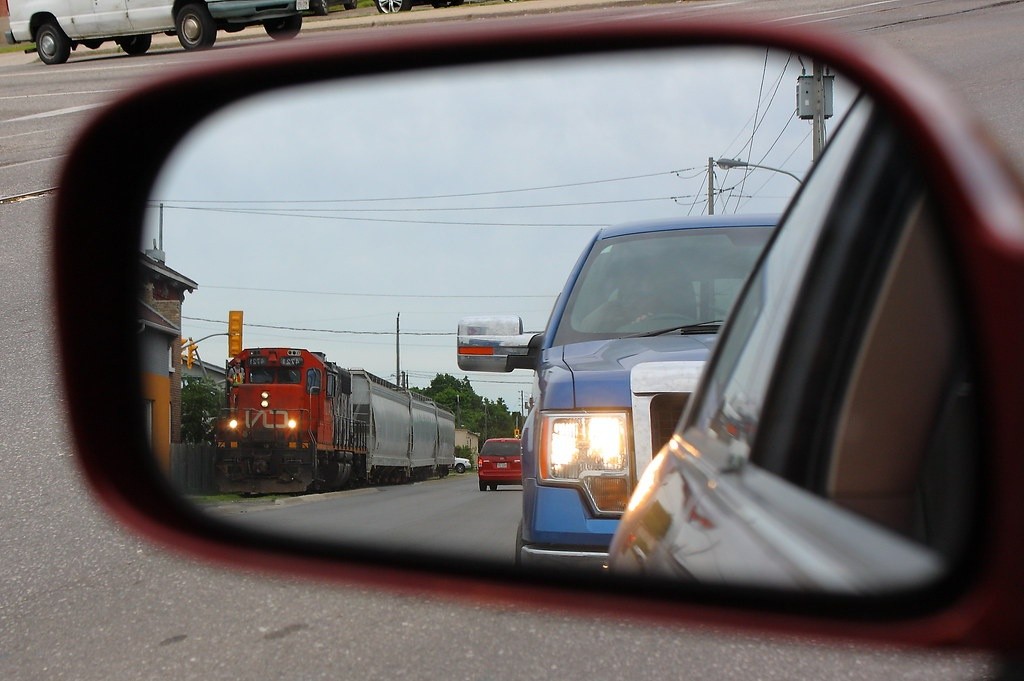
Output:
[448,457,471,473]
[8,0,467,65]
[478,438,522,491]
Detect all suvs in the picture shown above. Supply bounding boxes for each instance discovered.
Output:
[458,208,786,583]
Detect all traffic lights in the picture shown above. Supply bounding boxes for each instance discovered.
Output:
[514,430,519,439]
[188,345,198,369]
[180,337,188,363]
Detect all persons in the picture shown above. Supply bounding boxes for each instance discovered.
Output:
[581,240,697,332]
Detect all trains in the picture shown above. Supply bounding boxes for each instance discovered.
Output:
[215,346,456,498]
